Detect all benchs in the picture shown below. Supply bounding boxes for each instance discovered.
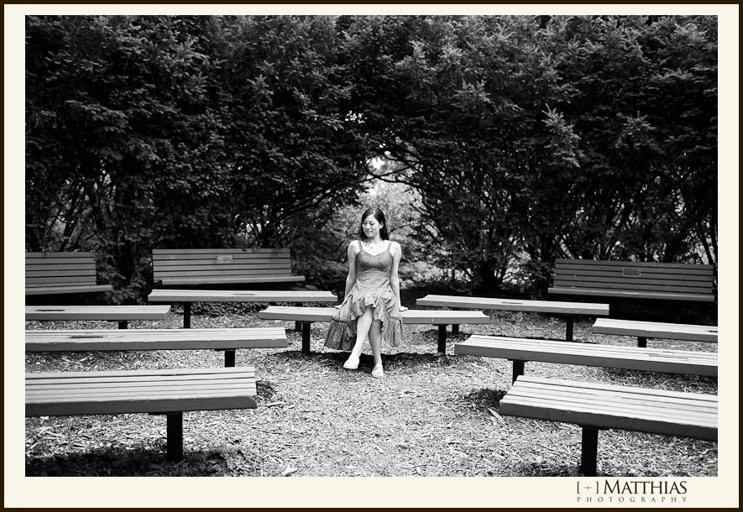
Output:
[24,244,717,476]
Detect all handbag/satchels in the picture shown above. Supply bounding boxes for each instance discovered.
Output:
[324,317,356,351]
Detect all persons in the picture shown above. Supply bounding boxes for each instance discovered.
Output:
[324,207,408,379]
